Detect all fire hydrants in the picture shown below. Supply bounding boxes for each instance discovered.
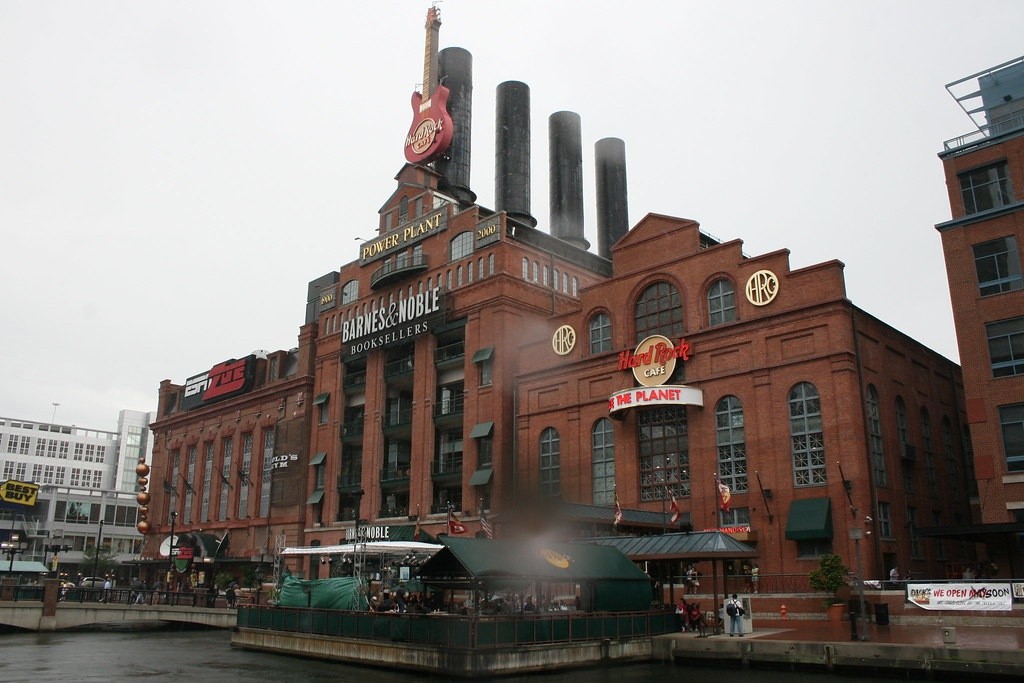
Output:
[780,605,787,621]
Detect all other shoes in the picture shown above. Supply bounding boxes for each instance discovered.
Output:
[682,627,685,632]
[739,634,743,637]
[730,633,734,637]
[753,591,758,594]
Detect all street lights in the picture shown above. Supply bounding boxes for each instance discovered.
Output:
[165,511,178,604]
[92,517,106,587]
[0,534,28,578]
[42,528,73,572]
[849,528,869,641]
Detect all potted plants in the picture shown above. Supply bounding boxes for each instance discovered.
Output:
[808,553,852,621]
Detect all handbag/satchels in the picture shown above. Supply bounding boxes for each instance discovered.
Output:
[695,582,700,586]
[739,608,745,616]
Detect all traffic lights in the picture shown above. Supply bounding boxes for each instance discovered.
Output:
[169,572,172,584]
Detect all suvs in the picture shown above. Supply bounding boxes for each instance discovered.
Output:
[81,577,106,588]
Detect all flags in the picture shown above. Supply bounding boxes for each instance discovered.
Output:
[665,484,680,525]
[413,514,420,541]
[448,513,468,534]
[714,478,734,513]
[479,504,495,539]
[612,491,623,526]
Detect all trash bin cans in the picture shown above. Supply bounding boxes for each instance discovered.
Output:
[873,602,889,625]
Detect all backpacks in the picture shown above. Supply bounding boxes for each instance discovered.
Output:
[727,600,736,616]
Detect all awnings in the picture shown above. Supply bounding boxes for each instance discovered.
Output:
[1005,502,1024,509]
[785,497,831,539]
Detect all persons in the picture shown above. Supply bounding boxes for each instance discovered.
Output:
[717,604,725,631]
[394,584,406,613]
[962,566,975,582]
[677,598,687,632]
[726,593,745,637]
[743,564,752,594]
[751,563,760,594]
[225,583,240,609]
[890,565,899,582]
[135,580,147,604]
[98,577,112,602]
[683,564,698,594]
[689,603,702,631]
[383,583,390,601]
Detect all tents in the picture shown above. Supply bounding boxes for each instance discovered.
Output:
[548,530,760,635]
[391,578,434,591]
[410,532,653,611]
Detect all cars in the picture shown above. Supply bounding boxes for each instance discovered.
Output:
[42,579,76,588]
